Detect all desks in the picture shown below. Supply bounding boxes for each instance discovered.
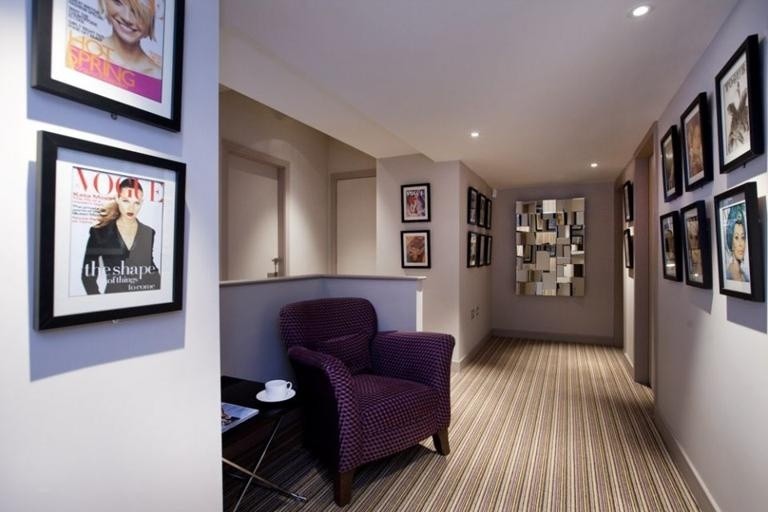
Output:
[221,376,308,512]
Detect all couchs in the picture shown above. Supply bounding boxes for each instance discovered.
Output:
[280,297,455,507]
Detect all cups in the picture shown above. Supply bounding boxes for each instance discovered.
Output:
[265,379,293,396]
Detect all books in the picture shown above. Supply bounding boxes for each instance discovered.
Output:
[221,401,260,433]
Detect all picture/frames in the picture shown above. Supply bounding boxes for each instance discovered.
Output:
[679,91,714,192]
[34,131,186,331]
[660,124,683,203]
[715,33,764,174]
[623,228,633,268]
[400,182,431,222]
[623,180,633,222]
[659,211,682,283]
[713,182,765,302]
[31,0,186,133]
[400,230,431,269]
[466,187,493,268]
[680,200,712,289]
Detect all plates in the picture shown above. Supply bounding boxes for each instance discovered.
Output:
[256,389,296,403]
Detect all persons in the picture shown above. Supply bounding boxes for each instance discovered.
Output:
[721,204,751,282]
[87,0,162,81]
[82,177,161,294]
[683,216,704,274]
